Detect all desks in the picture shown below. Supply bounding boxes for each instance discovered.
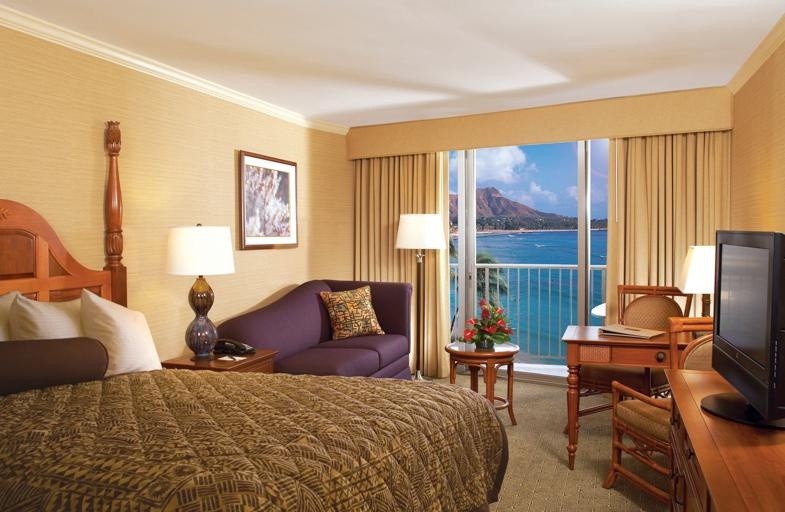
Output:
[561,325,718,469]
[445,341,521,424]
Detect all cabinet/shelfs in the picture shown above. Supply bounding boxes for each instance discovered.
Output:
[659,368,785,512]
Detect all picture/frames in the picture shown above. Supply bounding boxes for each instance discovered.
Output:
[235,150,298,250]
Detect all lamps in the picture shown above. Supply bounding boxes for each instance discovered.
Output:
[678,246,716,318]
[167,224,236,361]
[394,214,447,383]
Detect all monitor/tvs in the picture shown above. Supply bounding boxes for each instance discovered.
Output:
[700,229,785,431]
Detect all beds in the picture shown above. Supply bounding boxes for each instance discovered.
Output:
[0,122,510,511]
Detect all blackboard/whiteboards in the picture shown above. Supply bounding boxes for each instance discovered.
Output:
[212,339,255,356]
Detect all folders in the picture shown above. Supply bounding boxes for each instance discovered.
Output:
[598,323,667,340]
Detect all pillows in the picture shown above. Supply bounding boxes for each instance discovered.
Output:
[0,338,109,395]
[9,293,83,339]
[319,285,386,341]
[80,287,162,378]
[0,291,20,338]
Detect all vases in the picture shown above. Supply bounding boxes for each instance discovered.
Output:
[476,342,495,349]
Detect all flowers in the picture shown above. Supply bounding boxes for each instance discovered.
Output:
[459,300,514,344]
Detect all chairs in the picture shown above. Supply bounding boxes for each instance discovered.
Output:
[602,317,719,502]
[617,285,693,398]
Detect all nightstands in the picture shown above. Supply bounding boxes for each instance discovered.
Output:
[161,351,277,372]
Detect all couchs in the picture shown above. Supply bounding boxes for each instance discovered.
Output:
[217,279,413,381]
